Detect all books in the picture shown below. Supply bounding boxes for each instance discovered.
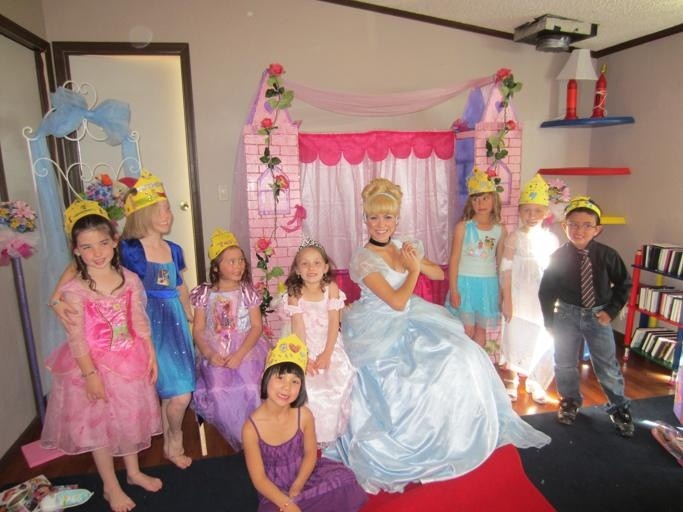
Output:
[629,243,682,362]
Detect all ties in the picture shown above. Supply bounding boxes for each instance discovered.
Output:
[577,248,596,308]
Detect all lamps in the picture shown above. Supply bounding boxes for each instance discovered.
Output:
[554,48,598,120]
[512,14,598,52]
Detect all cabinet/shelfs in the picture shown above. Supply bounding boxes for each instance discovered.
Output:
[537,117,635,224]
[622,249,683,378]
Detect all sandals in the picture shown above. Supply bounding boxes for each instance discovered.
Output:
[525,378,546,405]
[503,376,519,402]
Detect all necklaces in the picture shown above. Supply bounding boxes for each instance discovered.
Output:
[368,237,391,247]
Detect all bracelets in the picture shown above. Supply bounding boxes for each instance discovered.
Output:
[48,296,63,308]
[80,368,96,378]
[279,502,289,512]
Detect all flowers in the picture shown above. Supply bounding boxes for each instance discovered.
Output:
[0,200,38,233]
[250,62,293,338]
[484,68,524,193]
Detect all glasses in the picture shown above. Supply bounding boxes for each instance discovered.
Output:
[565,222,597,230]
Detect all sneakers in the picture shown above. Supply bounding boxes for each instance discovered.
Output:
[559,397,579,424]
[610,407,635,436]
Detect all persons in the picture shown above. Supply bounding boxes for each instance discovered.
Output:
[47,169,191,470]
[38,201,164,511]
[281,236,353,445]
[537,194,636,438]
[498,172,559,404]
[188,227,274,450]
[442,169,508,348]
[241,330,365,512]
[320,177,551,494]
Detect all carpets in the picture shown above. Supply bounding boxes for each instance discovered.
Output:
[0,394,683,512]
